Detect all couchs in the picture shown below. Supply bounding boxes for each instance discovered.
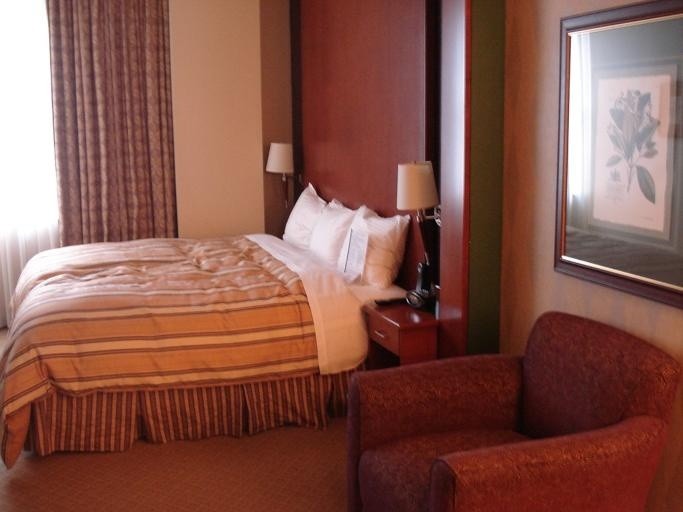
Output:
[16,232,410,460]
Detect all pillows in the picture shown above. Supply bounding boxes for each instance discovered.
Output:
[280,181,411,282]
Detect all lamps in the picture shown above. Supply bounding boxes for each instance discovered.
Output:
[265,140,303,188]
[396,156,443,227]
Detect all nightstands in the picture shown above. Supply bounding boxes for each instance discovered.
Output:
[361,303,439,366]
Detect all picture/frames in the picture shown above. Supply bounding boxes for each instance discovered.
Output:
[552,2,683,310]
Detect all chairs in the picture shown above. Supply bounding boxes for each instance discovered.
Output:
[349,305,683,512]
